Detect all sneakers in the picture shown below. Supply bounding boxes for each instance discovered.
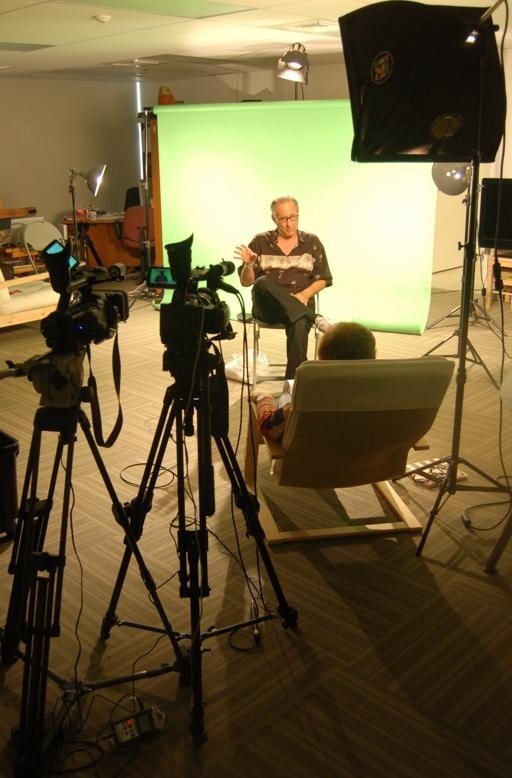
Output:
[314,313,332,333]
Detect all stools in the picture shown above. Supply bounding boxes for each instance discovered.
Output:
[252,290,320,393]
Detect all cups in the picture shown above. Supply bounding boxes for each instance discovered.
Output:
[88,210,97,218]
[76,208,83,217]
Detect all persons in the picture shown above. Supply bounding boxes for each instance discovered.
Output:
[234,197,333,381]
[247,322,377,443]
[155,269,168,283]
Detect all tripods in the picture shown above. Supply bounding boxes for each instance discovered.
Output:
[1,409,181,778]
[94,378,300,751]
[425,187,507,337]
[418,183,500,391]
[391,139,512,556]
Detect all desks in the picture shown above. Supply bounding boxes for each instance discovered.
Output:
[62,209,155,269]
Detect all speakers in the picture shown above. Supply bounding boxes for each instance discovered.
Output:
[478,178,512,251]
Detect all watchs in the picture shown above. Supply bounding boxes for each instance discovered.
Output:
[256,395,272,402]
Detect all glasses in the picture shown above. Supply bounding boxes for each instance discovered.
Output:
[275,213,298,223]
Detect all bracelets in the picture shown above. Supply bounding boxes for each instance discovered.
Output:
[245,263,253,269]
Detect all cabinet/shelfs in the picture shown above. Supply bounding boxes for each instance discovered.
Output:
[484,249,511,312]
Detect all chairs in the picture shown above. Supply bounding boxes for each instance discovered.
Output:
[23,218,67,274]
[114,204,156,286]
[0,267,63,330]
[243,354,457,549]
[123,185,141,210]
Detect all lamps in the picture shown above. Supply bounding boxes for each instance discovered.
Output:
[274,40,311,101]
[418,162,502,389]
[336,0,512,564]
[64,160,110,267]
